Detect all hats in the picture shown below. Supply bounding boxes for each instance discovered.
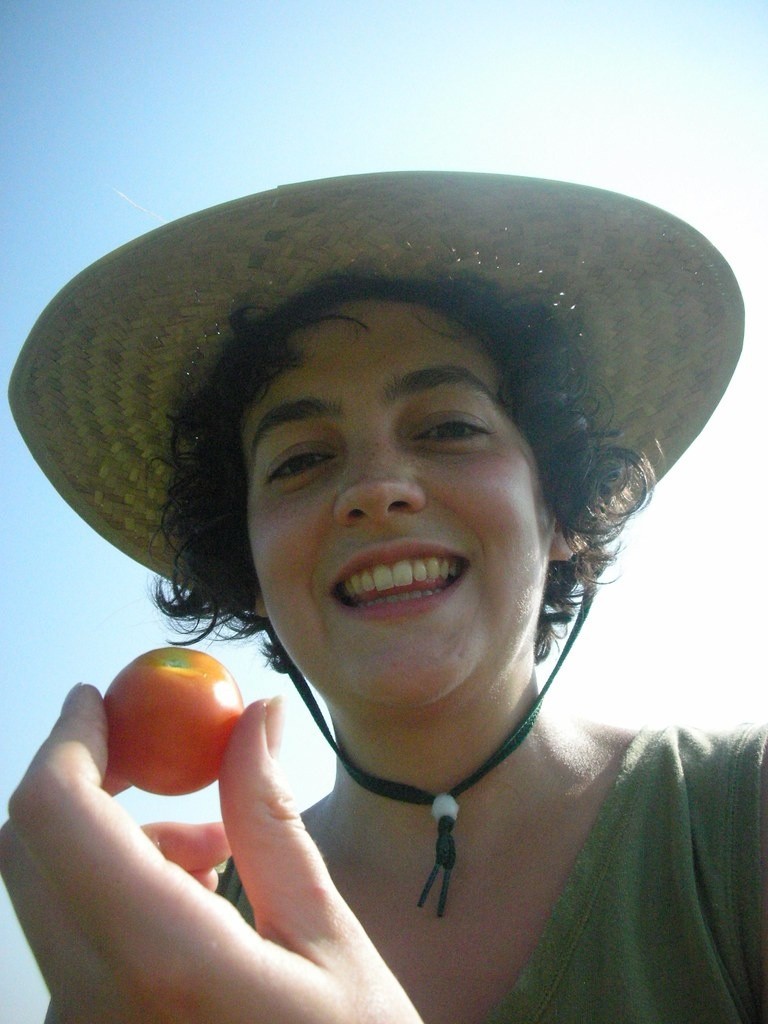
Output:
[7,173,745,591]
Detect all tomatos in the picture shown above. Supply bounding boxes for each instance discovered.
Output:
[101,648,244,795]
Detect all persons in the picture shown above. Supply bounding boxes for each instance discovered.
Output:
[1,169,768,1024]
